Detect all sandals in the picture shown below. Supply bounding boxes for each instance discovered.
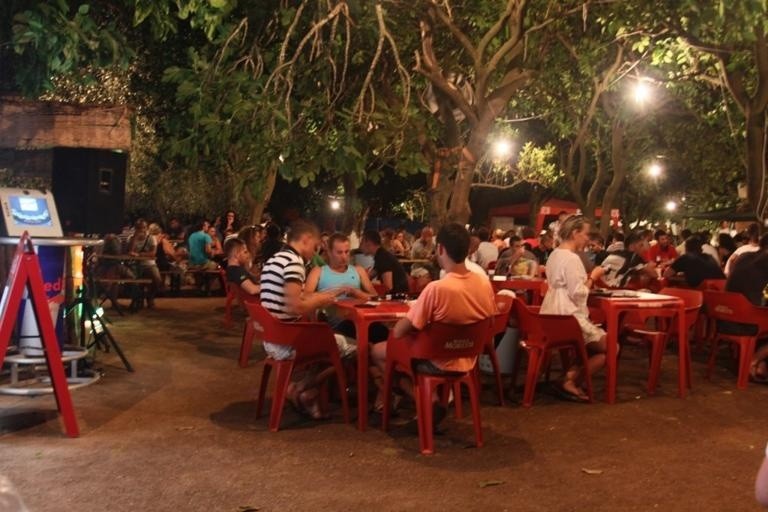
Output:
[287,380,321,421]
[549,377,589,403]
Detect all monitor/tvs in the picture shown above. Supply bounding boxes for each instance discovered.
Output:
[0,187,64,238]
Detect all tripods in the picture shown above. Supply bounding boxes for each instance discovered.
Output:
[64,233,136,373]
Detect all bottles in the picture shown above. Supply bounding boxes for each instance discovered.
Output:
[655,255,663,280]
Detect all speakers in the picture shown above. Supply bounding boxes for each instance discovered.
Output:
[51,146,129,234]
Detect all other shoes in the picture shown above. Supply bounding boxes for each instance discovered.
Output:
[373,400,385,411]
[391,394,403,410]
[409,400,447,433]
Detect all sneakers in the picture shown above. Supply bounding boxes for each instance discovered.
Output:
[750,357,768,384]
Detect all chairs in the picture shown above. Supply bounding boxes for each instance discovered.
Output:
[381,315,492,455]
[221,239,768,432]
[81,240,228,317]
